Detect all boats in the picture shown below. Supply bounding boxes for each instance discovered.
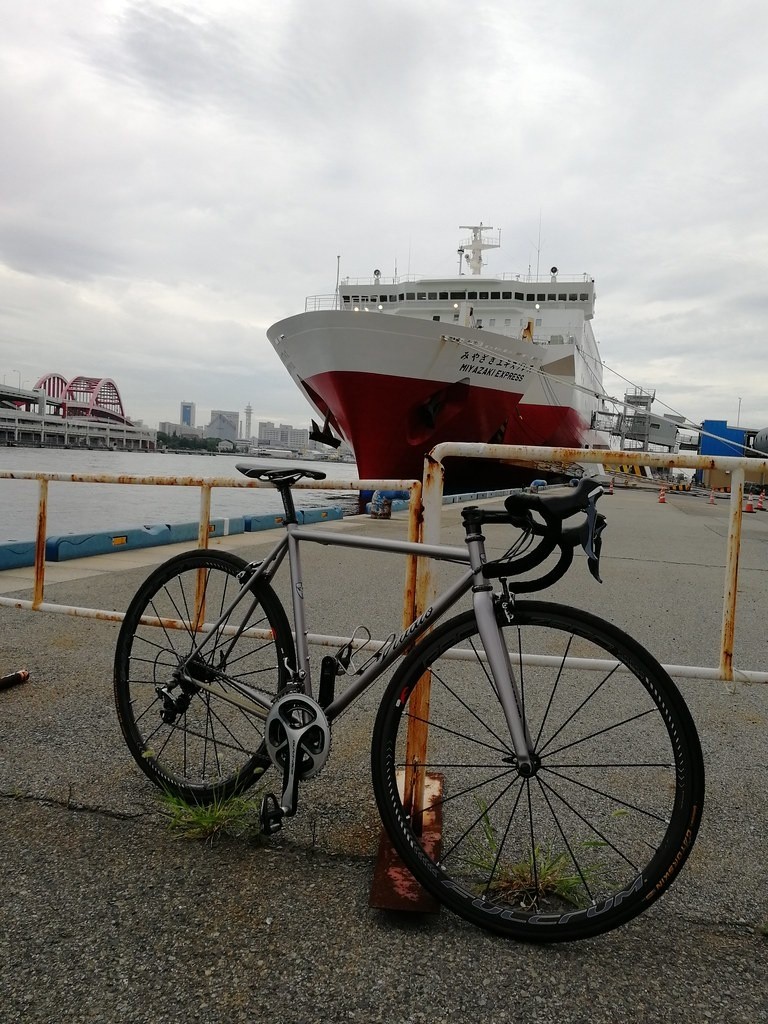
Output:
[263,216,701,516]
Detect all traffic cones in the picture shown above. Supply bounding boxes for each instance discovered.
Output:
[742,491,756,513]
[753,492,767,511]
[658,485,667,503]
[608,479,614,494]
[706,487,717,506]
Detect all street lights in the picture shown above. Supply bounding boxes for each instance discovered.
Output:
[737,395,742,427]
[13,370,21,388]
[4,374,8,385]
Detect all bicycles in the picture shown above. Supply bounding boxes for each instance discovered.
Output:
[111,464,706,947]
